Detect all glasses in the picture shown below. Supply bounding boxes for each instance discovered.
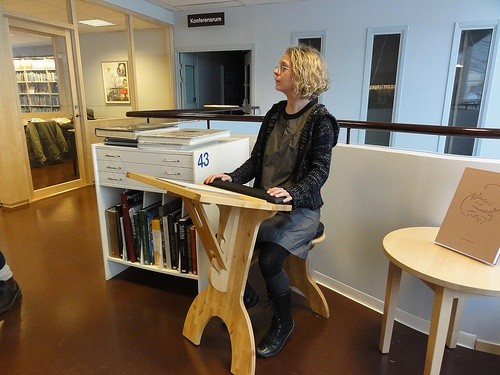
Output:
[276,64,292,72]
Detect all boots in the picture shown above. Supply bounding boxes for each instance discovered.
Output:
[243,279,259,309]
[255,284,296,358]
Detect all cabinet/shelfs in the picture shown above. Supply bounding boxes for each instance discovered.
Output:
[12,57,61,113]
[90,136,249,295]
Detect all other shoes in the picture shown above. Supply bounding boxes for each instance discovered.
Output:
[0,276,22,314]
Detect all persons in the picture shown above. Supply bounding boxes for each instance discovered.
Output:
[203,45,340,357]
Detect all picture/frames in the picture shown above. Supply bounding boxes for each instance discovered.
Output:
[101,60,131,105]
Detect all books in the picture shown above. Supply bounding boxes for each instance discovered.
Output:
[17,71,60,113]
[95,123,231,151]
[105,190,198,275]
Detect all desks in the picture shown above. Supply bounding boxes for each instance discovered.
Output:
[378,226,500,375]
